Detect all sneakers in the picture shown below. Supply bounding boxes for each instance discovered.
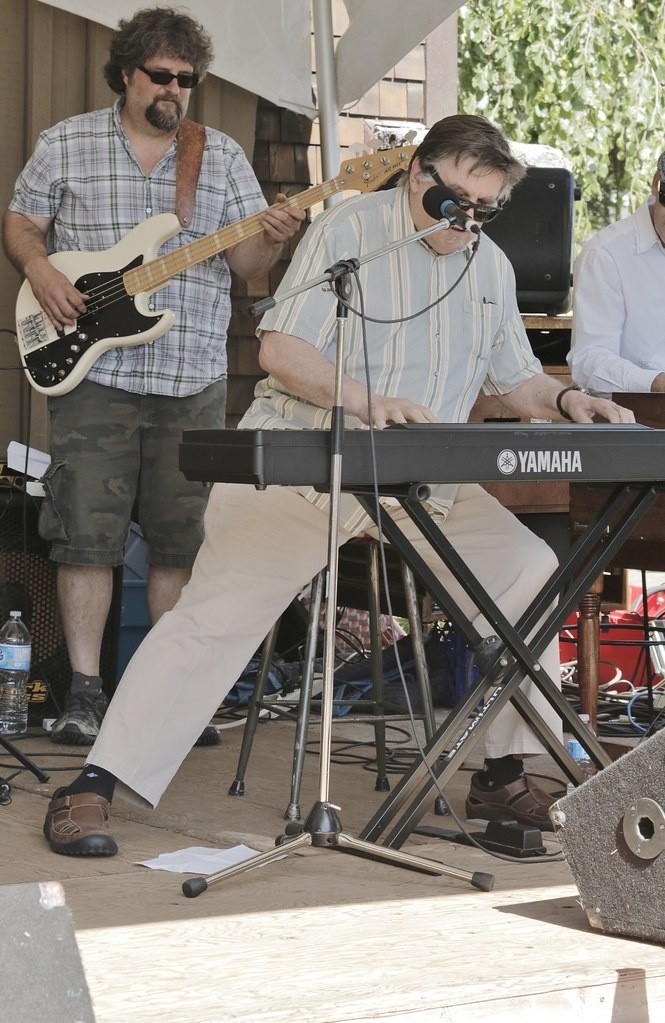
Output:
[48,686,110,745]
[194,721,221,746]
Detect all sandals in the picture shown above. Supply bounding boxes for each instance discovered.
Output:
[465,770,559,832]
[42,785,119,857]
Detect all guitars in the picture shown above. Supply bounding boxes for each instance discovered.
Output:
[14,128,419,398]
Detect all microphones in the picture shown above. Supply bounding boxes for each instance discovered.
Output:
[422,185,481,234]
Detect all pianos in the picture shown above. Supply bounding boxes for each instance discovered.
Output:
[180,421,665,492]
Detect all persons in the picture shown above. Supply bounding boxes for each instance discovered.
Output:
[3,4,306,748]
[43,115,635,856]
[564,147,665,396]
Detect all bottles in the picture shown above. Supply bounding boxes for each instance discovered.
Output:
[569,713,596,791]
[0,611,31,733]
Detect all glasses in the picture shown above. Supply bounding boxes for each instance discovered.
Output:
[657,180,665,205]
[136,63,199,89]
[424,165,504,223]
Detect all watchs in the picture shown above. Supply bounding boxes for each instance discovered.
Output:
[556,383,590,421]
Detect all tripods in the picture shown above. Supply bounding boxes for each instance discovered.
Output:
[182,213,495,899]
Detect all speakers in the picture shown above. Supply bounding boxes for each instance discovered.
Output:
[0,485,124,729]
[0,882,96,1023]
[548,724,665,944]
[368,126,581,318]
